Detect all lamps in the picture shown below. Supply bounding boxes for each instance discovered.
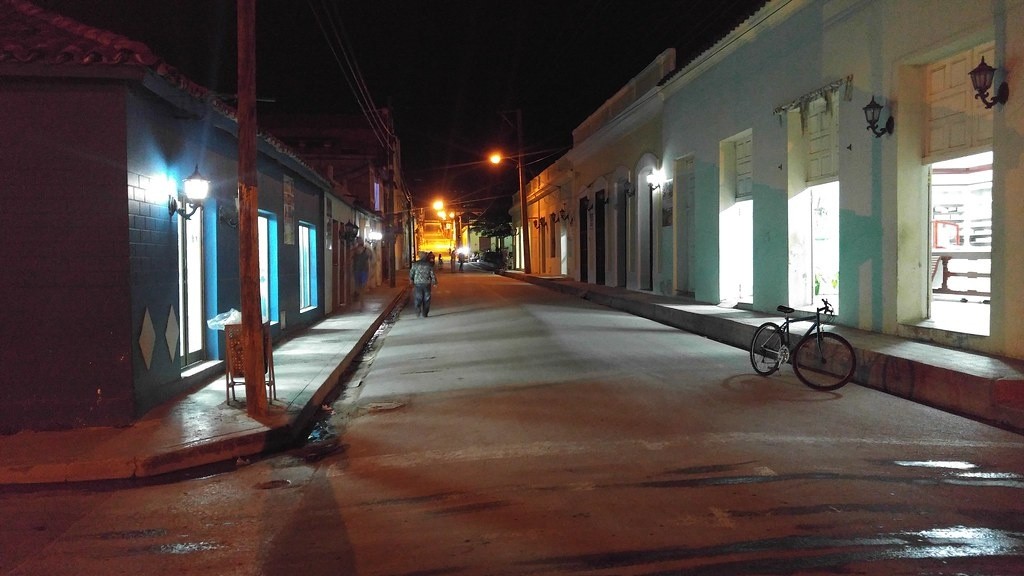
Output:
[539,218,547,226]
[969,57,1009,109]
[863,96,894,138]
[550,213,559,223]
[583,196,593,211]
[166,164,210,220]
[220,192,239,228]
[534,220,539,229]
[624,181,635,197]
[339,220,359,240]
[560,209,569,220]
[598,190,609,204]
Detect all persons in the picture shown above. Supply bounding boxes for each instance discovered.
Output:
[409,251,438,318]
[457,252,465,272]
[348,239,370,307]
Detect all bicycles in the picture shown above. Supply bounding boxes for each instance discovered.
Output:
[749,292,858,390]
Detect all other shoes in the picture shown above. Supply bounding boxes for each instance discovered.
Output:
[423,313,427,317]
[416,309,421,316]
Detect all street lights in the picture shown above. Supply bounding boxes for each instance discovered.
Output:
[489,153,533,276]
